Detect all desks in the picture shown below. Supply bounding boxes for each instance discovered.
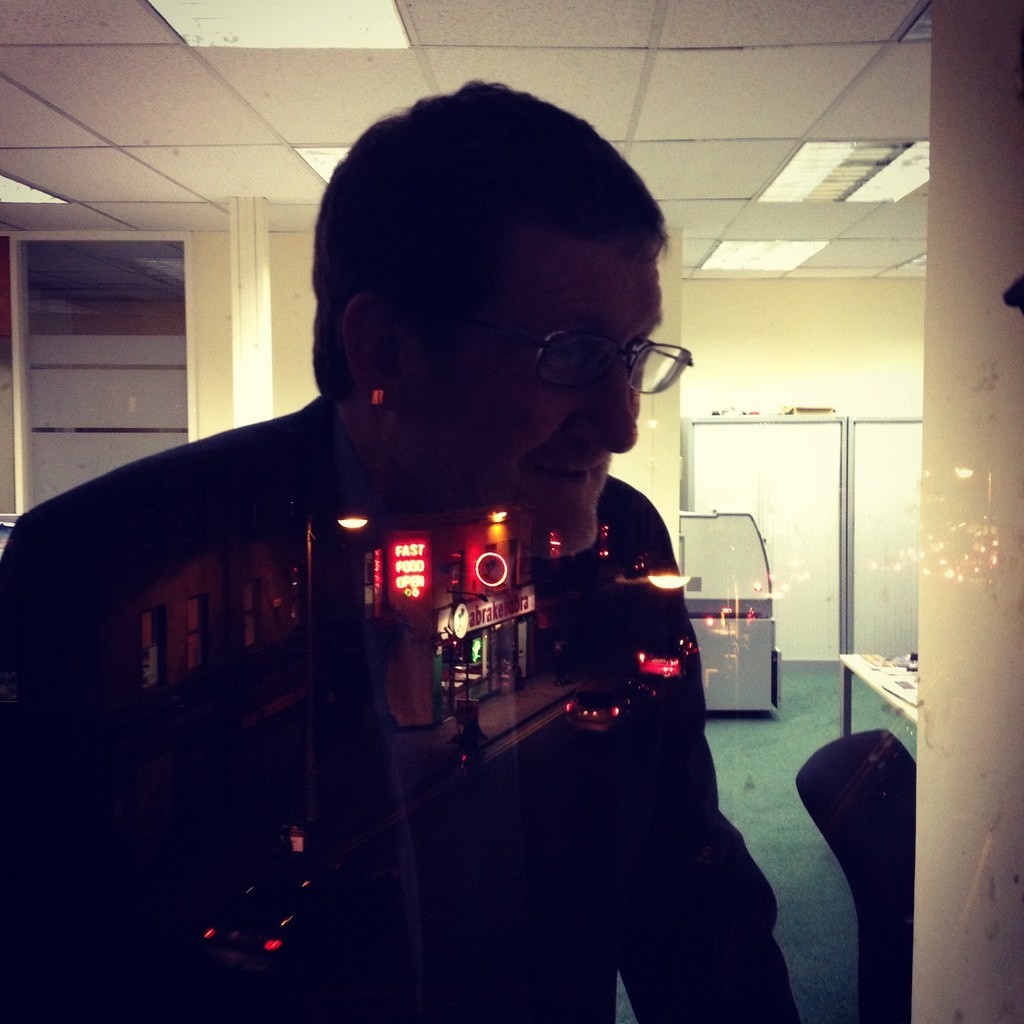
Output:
[839,652,916,734]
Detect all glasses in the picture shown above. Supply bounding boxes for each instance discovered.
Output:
[445,314,693,395]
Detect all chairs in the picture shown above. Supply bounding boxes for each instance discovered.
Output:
[796,728,916,1024]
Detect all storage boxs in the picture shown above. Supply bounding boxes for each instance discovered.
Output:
[783,407,835,414]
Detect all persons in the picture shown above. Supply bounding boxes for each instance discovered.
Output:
[0,80,801,1024]
